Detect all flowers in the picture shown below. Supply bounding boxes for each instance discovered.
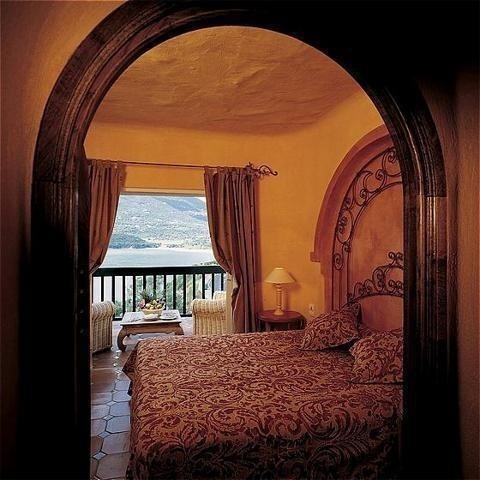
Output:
[136,289,168,309]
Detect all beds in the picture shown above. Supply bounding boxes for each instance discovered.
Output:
[120,124,405,479]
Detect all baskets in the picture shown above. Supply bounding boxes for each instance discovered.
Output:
[140,307,165,316]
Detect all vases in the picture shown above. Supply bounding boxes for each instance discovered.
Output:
[141,309,163,317]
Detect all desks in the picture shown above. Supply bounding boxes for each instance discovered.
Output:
[117,308,185,353]
[254,308,304,333]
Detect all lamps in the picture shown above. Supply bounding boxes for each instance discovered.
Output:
[263,266,297,316]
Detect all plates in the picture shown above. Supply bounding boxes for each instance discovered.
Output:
[144,316,158,320]
[160,309,178,320]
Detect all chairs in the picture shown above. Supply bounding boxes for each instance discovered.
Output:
[92,298,117,356]
[191,290,227,336]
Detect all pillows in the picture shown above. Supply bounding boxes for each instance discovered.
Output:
[347,327,405,385]
[299,300,363,352]
